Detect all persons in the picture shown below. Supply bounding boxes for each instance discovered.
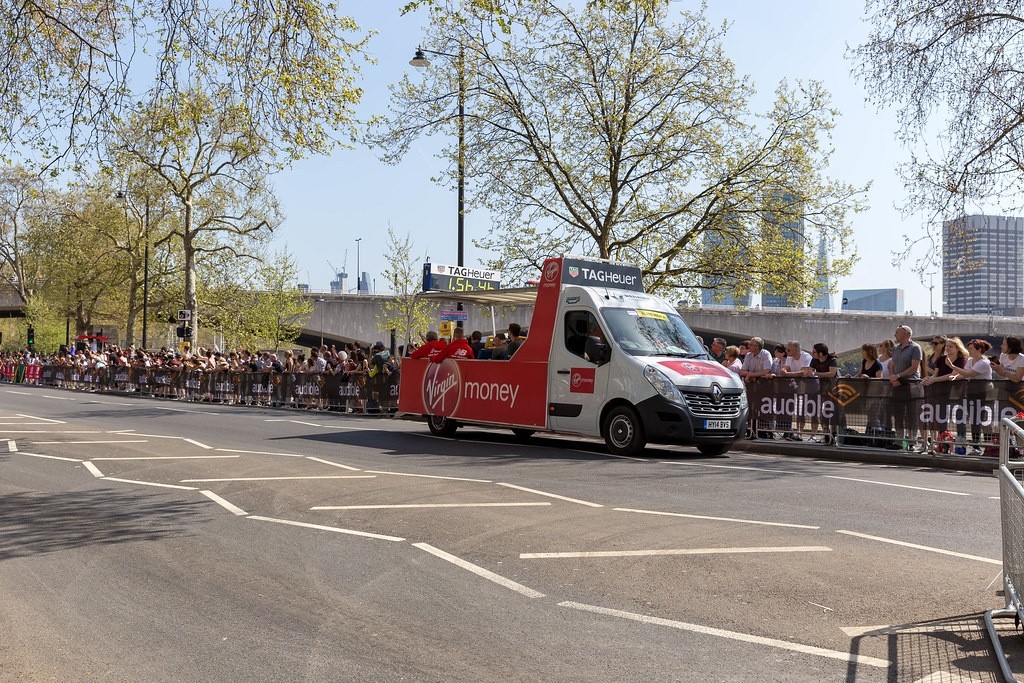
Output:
[696,325,1024,458]
[0,342,415,417]
[409,323,523,363]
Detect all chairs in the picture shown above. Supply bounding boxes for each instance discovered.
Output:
[491,348,506,360]
[567,319,588,355]
[504,341,522,360]
[476,349,492,359]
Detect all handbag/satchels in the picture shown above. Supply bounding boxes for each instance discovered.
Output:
[368,363,378,377]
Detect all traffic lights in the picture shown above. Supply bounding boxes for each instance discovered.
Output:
[185,326,192,337]
[176,326,186,337]
[27,328,34,344]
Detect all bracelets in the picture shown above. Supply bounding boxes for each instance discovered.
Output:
[897,374,900,378]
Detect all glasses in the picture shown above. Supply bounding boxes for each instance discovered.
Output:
[749,343,758,346]
[740,347,746,349]
[880,345,883,348]
[972,339,982,347]
[931,341,942,345]
[813,351,818,354]
[788,349,791,352]
[899,325,906,332]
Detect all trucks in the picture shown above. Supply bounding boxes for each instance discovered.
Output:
[397,254,749,459]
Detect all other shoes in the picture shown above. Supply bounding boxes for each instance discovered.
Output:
[747,430,1024,460]
[5,379,414,416]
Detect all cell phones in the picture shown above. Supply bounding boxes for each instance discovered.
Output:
[989,356,1000,366]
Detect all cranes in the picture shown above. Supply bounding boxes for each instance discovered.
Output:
[325,258,342,281]
[340,249,348,274]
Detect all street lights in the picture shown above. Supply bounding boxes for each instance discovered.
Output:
[409,40,466,328]
[926,272,936,315]
[355,238,362,289]
[115,191,149,349]
